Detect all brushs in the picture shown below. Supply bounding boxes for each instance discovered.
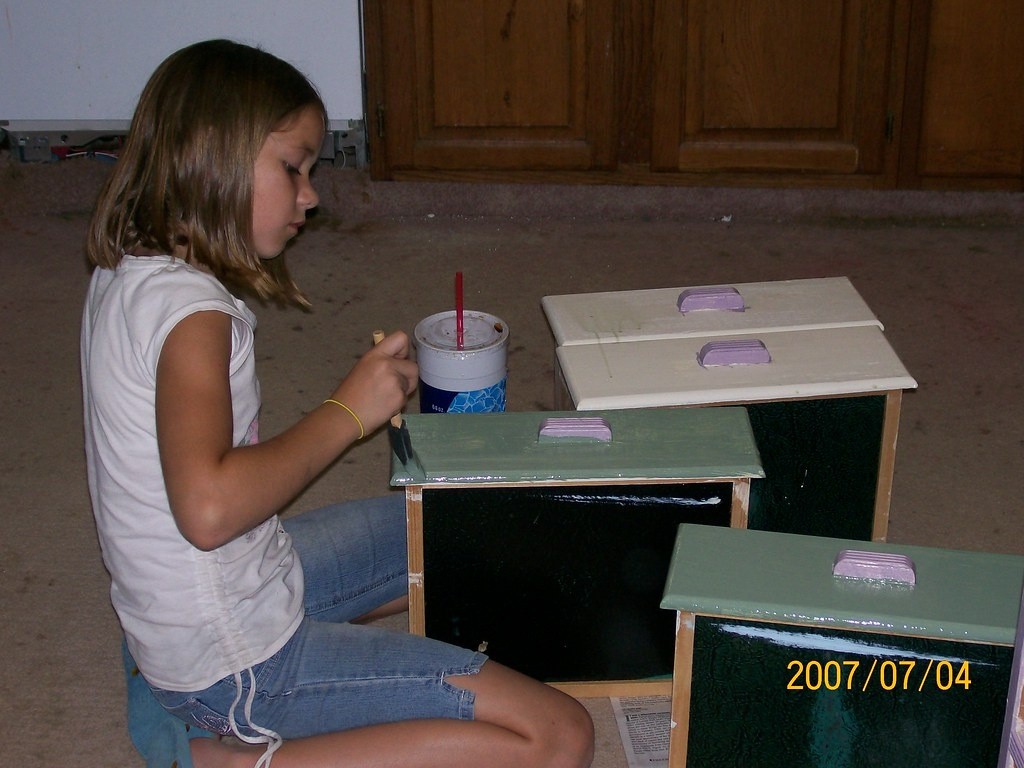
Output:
[371,329,427,484]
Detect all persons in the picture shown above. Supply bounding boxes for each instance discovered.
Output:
[79,39,594,768]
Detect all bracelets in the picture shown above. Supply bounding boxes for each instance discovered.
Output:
[324,399,364,439]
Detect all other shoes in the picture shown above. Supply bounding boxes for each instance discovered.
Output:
[122,633,217,768]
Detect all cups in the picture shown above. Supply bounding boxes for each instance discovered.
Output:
[414,310,509,413]
